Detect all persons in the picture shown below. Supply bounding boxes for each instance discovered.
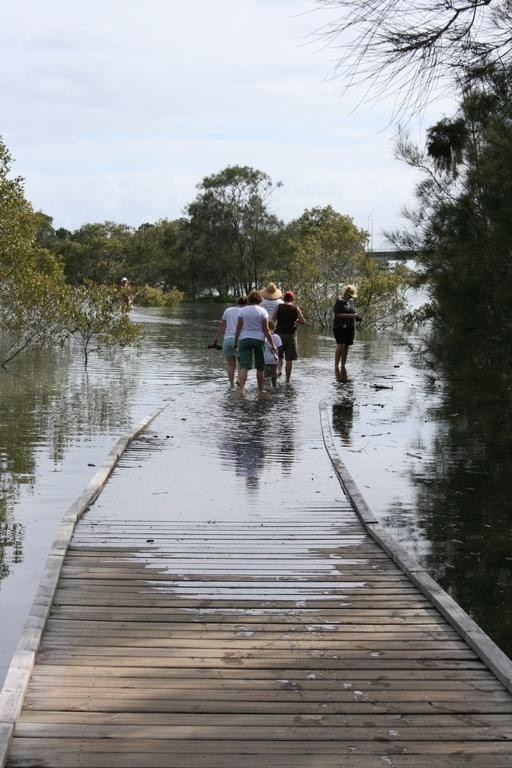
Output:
[331,285,362,381]
[213,297,248,390]
[233,290,277,394]
[269,292,308,384]
[257,282,284,379]
[263,320,283,390]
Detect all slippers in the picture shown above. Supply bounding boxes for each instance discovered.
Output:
[232,345,239,351]
[269,347,278,362]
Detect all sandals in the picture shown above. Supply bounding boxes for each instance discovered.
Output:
[208,343,222,350]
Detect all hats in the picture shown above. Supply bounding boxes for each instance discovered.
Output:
[121,277,128,283]
[343,285,357,298]
[268,320,276,330]
[260,283,282,299]
